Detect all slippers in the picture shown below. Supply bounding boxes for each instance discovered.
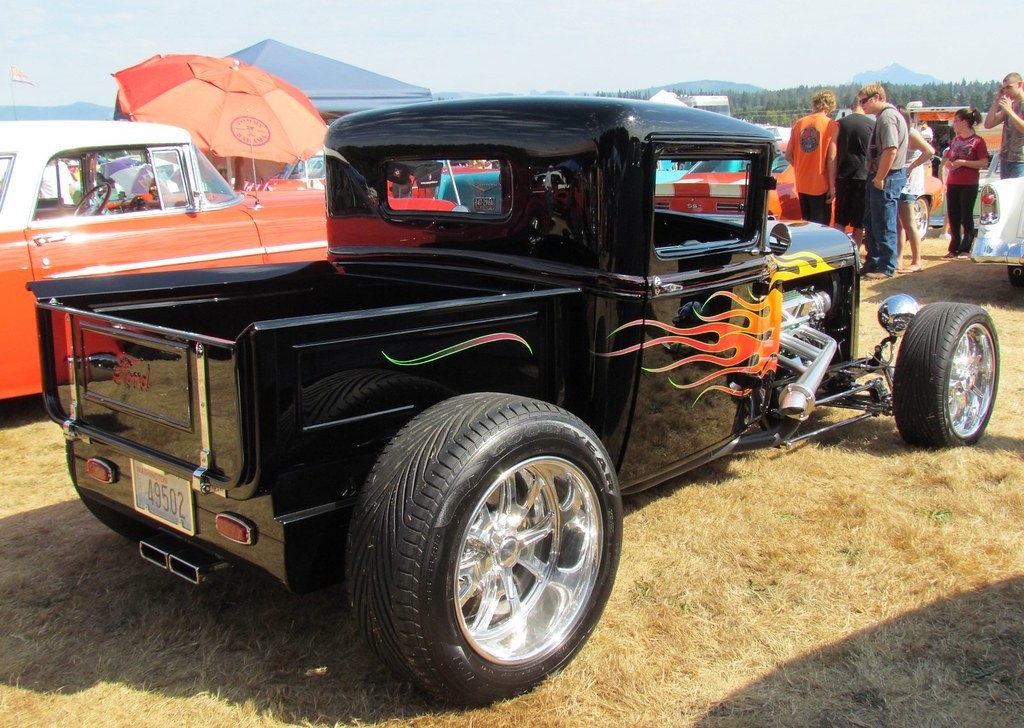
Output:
[897,266,924,276]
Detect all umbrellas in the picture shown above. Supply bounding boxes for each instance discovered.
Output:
[100,158,168,195]
[110,55,331,196]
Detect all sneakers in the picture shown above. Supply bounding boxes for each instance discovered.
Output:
[942,252,958,262]
[955,251,971,261]
[865,271,892,279]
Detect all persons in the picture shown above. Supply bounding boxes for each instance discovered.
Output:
[786,90,841,227]
[944,108,992,261]
[833,95,878,273]
[218,163,228,189]
[38,155,79,207]
[154,161,180,195]
[895,106,938,275]
[940,138,978,242]
[857,83,910,281]
[66,159,105,183]
[985,74,1024,181]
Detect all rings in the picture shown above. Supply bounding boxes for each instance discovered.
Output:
[907,174,910,177]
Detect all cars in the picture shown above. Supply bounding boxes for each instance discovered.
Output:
[437,153,502,213]
[1,116,477,401]
[969,150,1024,292]
[923,145,1001,229]
[654,116,946,239]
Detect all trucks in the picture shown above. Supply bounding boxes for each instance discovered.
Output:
[682,92,731,118]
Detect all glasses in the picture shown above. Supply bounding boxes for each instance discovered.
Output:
[860,95,874,103]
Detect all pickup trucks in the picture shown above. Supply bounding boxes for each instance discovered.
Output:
[25,93,1000,715]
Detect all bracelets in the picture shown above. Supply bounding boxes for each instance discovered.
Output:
[875,178,884,186]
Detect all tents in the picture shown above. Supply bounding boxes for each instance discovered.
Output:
[115,41,435,194]
